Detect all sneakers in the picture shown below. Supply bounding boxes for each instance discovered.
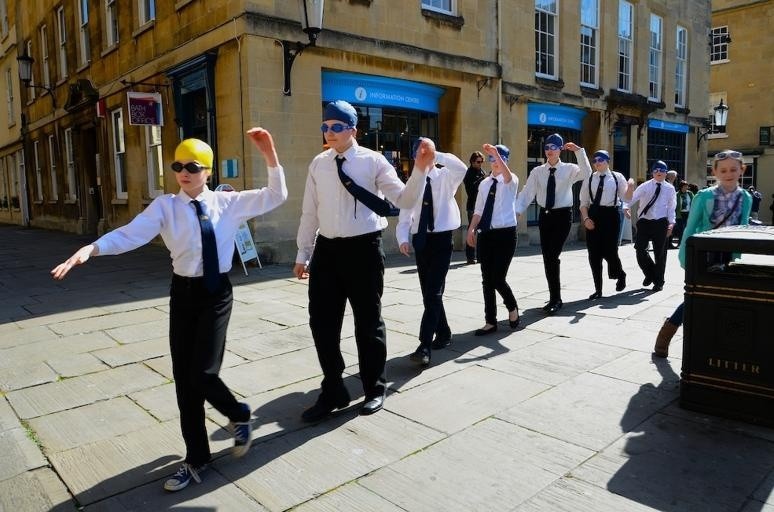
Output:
[164,462,207,491]
[232,403,251,456]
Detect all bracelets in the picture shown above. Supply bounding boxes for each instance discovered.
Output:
[582,217,589,222]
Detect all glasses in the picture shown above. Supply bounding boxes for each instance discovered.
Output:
[171,162,210,174]
[474,161,483,164]
[321,123,355,133]
[654,170,665,173]
[715,152,741,160]
[544,145,559,150]
[591,159,604,163]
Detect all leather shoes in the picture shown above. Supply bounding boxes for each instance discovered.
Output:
[466,257,475,264]
[361,392,386,414]
[302,395,350,423]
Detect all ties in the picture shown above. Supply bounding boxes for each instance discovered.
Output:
[414,177,434,250]
[546,167,556,210]
[588,175,605,218]
[639,183,661,217]
[192,201,219,295]
[477,178,497,232]
[335,155,400,216]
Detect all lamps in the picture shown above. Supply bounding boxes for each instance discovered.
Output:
[17,56,55,105]
[277,0,325,97]
[697,98,729,152]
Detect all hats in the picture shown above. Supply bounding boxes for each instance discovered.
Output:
[593,149,609,160]
[544,134,563,150]
[489,144,509,161]
[323,100,359,127]
[175,138,213,174]
[652,160,667,170]
[412,137,422,158]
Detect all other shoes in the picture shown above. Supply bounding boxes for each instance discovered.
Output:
[475,324,497,337]
[643,278,655,286]
[653,285,663,291]
[410,346,431,364]
[615,277,627,291]
[544,299,563,314]
[509,307,520,328]
[431,329,452,349]
[589,292,602,299]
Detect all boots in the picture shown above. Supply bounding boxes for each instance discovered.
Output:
[655,317,679,357]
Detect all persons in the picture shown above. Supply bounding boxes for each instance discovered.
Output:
[466,143,521,336]
[664,170,679,249]
[579,149,635,301]
[293,100,437,425]
[654,149,753,358]
[48,128,290,495]
[616,197,631,246]
[747,186,762,220]
[625,159,677,293]
[395,135,471,366]
[514,133,593,314]
[464,150,493,265]
[676,180,713,249]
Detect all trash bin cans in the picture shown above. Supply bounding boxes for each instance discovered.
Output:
[678,225,774,429]
